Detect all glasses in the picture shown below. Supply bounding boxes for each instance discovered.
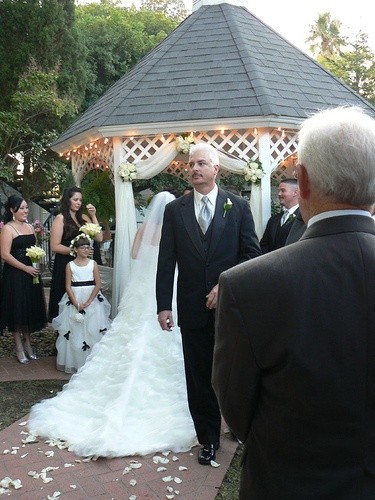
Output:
[78,246,92,252]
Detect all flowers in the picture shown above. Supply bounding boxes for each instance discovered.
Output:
[26,245,46,284]
[0,219,50,247]
[175,135,197,154]
[243,161,267,183]
[69,233,94,257]
[286,213,296,225]
[79,222,102,259]
[119,163,138,183]
[222,197,233,218]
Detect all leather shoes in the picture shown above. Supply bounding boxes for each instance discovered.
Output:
[198,441,220,465]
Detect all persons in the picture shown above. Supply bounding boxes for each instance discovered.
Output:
[209,103,375,500]
[156,142,262,465]
[47,185,103,356]
[51,232,113,373]
[128,191,186,368]
[0,194,47,364]
[91,219,111,267]
[259,178,306,254]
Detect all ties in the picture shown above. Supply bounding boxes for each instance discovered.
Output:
[198,196,213,235]
[283,211,290,223]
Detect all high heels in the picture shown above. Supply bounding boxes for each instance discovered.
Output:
[16,347,30,365]
[25,343,40,360]
